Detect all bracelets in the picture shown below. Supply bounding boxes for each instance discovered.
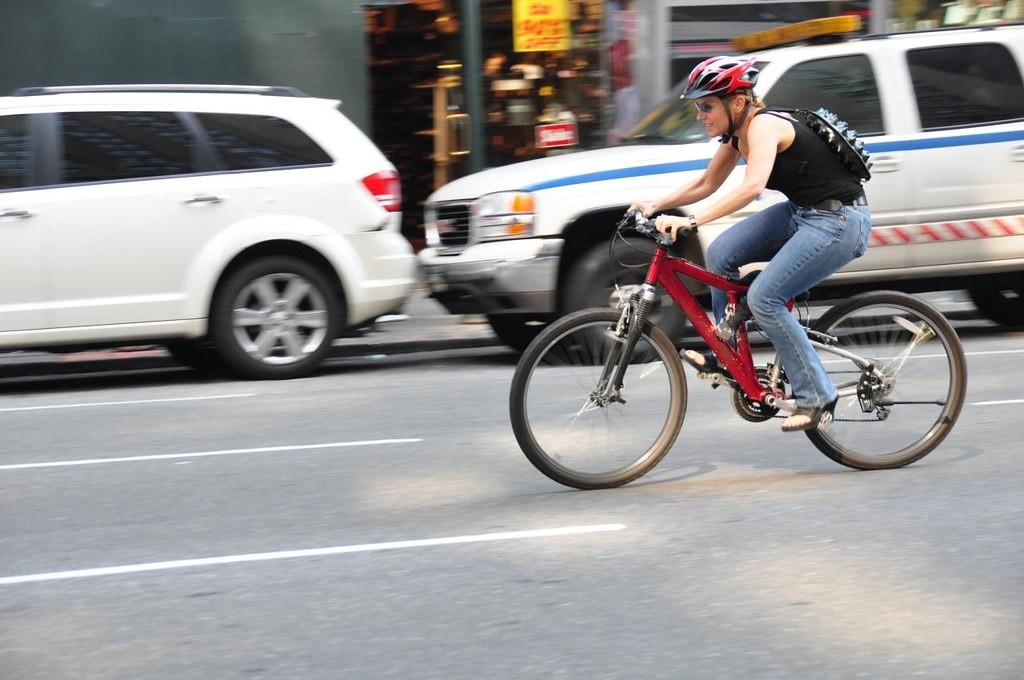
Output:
[689,215,697,233]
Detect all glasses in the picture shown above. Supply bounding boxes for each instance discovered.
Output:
[694,99,729,112]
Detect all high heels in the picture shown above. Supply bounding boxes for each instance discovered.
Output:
[781,394,838,432]
[680,349,734,389]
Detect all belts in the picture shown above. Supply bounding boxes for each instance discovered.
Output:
[803,194,867,212]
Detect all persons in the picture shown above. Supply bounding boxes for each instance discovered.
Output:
[627,56,873,432]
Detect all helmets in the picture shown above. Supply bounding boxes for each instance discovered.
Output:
[680,56,759,98]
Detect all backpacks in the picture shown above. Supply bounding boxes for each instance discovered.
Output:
[748,108,872,182]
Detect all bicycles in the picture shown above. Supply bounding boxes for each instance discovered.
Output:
[508,207,969,491]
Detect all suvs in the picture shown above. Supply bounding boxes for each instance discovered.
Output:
[416,16,1024,366]
[0,82,420,382]
